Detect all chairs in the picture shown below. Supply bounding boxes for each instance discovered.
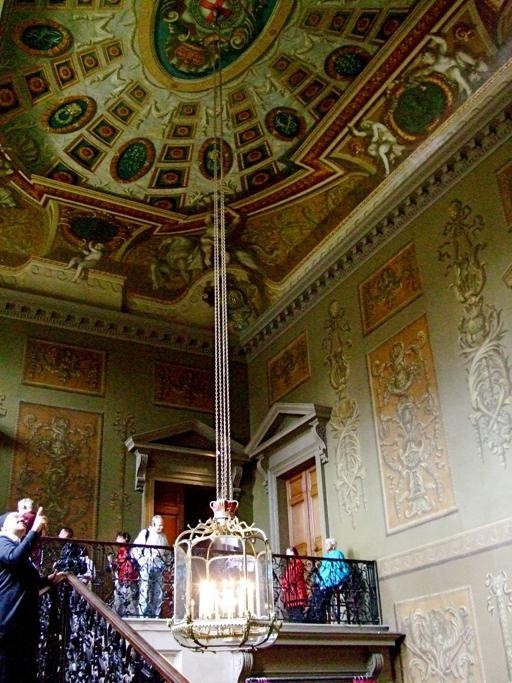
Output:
[327,563,366,625]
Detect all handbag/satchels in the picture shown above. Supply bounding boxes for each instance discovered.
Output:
[130,550,144,567]
[119,560,141,587]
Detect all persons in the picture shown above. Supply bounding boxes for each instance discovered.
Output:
[128,515,172,618]
[271,539,350,625]
[0,497,96,683]
[346,118,397,177]
[196,206,242,263]
[103,531,140,618]
[415,33,487,95]
[64,239,105,282]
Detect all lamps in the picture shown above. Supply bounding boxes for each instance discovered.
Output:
[172,7,280,652]
